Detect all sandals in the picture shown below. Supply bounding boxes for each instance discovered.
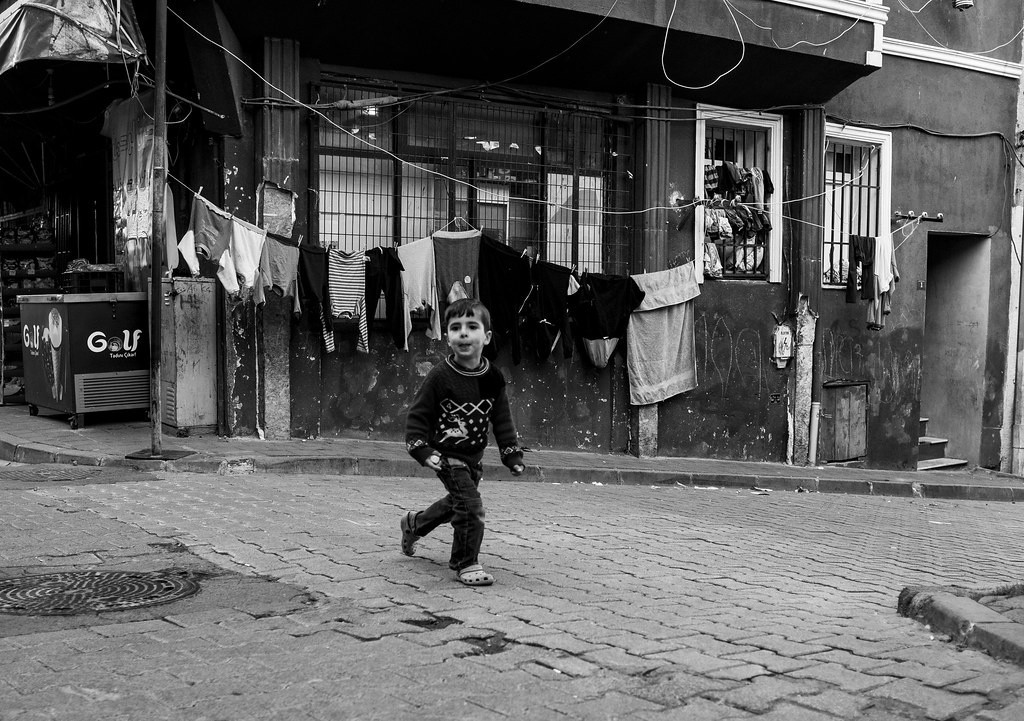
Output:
[400,509,425,556]
[457,563,494,585]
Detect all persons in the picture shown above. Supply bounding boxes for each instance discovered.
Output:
[400,299,526,585]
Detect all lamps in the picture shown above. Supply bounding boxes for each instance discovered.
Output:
[363,105,381,121]
